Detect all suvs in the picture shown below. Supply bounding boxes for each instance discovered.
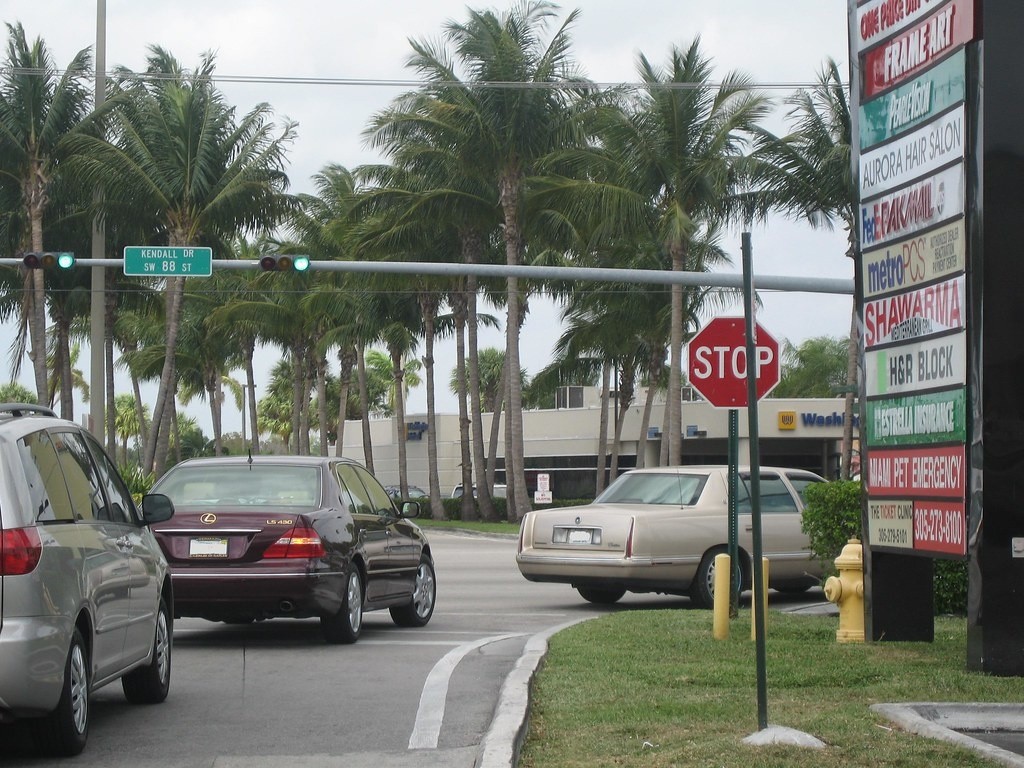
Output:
[2,401,178,758]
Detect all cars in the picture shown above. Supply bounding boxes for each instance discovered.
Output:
[129,452,438,644]
[382,484,429,500]
[451,483,478,500]
[513,461,838,610]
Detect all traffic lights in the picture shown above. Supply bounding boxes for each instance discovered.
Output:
[260,253,310,273]
[21,251,75,274]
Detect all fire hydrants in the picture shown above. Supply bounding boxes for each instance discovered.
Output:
[824,537,865,644]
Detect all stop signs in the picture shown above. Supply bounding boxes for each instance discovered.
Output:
[688,316,782,410]
